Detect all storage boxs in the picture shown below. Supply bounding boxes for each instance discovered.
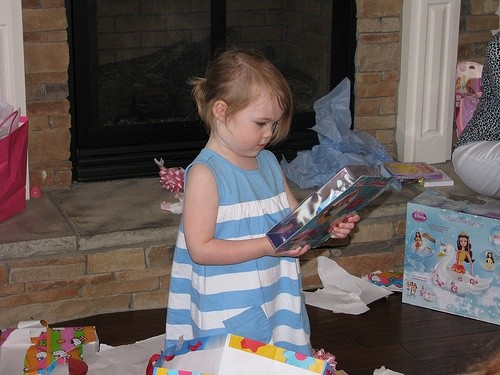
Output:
[401,190,500,323]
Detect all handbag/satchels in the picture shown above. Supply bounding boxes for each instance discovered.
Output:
[0,111,30,222]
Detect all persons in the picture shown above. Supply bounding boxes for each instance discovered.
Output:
[165,45,360,358]
[452,30,500,202]
